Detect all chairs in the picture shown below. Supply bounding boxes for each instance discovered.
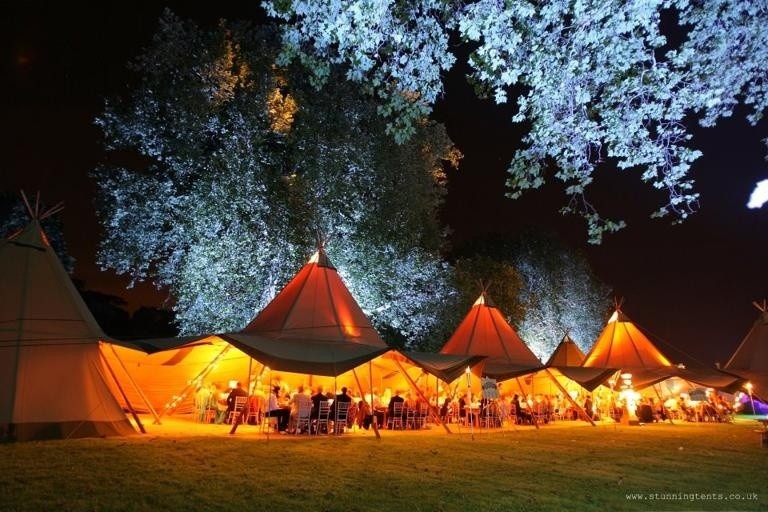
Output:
[203,393,520,429]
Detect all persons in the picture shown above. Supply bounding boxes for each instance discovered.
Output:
[194,380,736,435]
